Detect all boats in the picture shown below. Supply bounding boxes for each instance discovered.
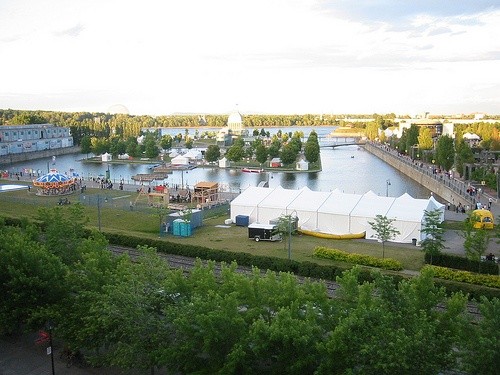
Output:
[242,168,264,173]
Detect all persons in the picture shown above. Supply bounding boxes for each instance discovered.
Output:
[368,135,494,216]
[0,165,206,206]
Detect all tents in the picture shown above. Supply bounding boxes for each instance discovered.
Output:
[229,183,447,248]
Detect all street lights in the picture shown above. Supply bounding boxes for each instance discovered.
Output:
[48,160,51,174]
[386,178,391,197]
[182,169,188,190]
